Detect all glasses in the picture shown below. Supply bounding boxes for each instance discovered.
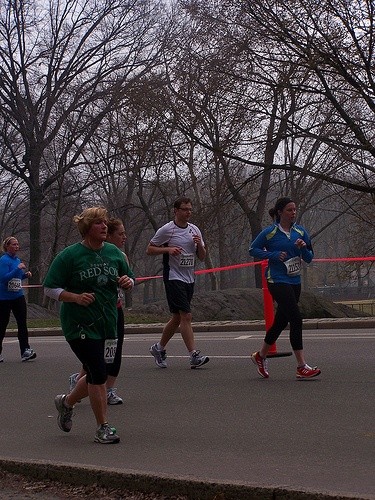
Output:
[176,207,192,211]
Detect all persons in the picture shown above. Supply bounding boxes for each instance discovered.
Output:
[249,197,322,379]
[146,198,209,368]
[42,208,135,443]
[68,218,129,405]
[0,236,37,362]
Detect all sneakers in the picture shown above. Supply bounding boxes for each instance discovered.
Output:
[69,373,79,390]
[94,422,120,443]
[107,388,124,405]
[250,351,270,378]
[296,364,322,378]
[55,394,75,432]
[21,351,37,363]
[190,350,209,368]
[148,343,168,368]
